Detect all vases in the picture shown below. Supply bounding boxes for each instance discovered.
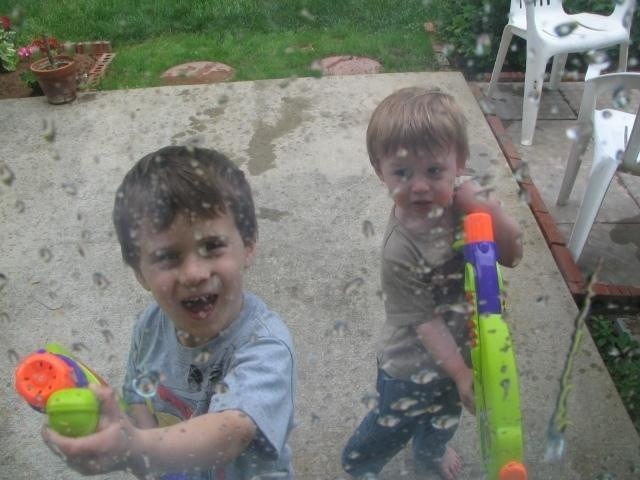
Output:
[30,58,78,106]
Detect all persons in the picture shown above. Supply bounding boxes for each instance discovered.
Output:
[43,145,299,479]
[341,87,524,480]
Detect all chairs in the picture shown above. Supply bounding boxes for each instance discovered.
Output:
[553,60,640,266]
[487,1,637,148]
